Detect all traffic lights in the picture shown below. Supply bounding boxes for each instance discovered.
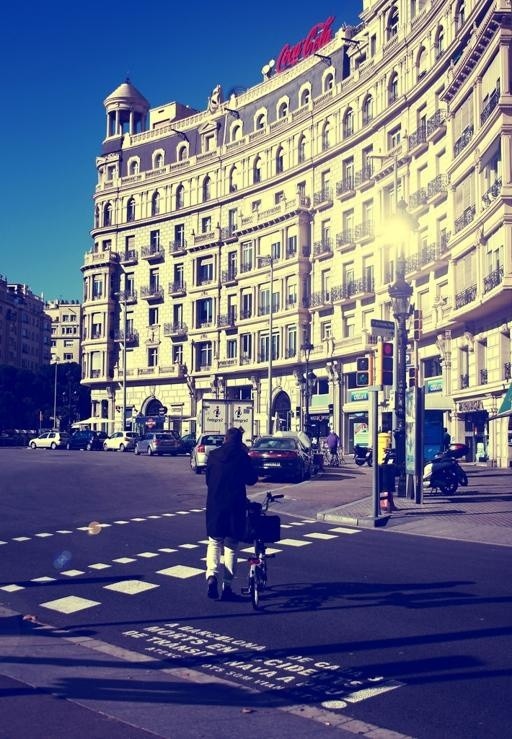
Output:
[355,357,370,386]
[377,343,394,385]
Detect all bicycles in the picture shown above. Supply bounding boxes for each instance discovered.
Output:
[323,446,344,467]
[241,491,286,610]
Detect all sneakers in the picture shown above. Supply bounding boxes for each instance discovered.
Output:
[208,576,219,599]
[221,582,233,601]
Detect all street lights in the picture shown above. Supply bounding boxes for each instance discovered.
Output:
[51,356,61,428]
[386,198,424,498]
[300,338,317,435]
[258,254,274,437]
[114,291,128,431]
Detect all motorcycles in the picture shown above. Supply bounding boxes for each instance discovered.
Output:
[352,443,372,466]
[382,442,470,495]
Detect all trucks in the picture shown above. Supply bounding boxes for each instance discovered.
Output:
[195,396,254,448]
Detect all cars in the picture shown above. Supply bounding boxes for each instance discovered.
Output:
[248,436,310,480]
[0,428,48,447]
[27,430,227,474]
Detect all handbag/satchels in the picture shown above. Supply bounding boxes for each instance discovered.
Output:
[240,513,280,543]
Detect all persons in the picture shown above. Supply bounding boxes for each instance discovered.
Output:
[204,428,259,601]
[444,427,451,451]
[327,429,340,465]
